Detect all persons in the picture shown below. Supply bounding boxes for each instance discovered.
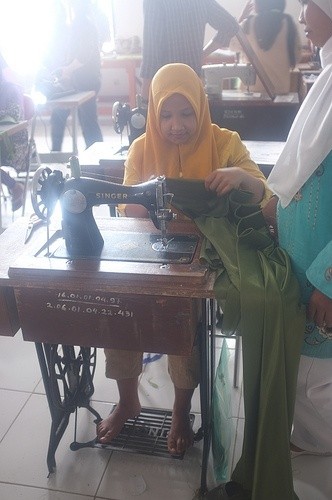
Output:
[267,0,332,500]
[142,1,238,85]
[0,75,28,211]
[98,62,265,457]
[42,1,106,152]
[235,1,299,93]
